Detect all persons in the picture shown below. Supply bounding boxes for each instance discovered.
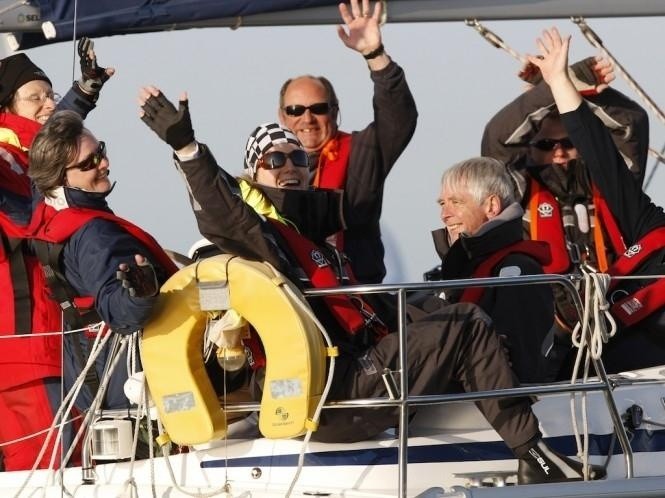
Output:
[279,1,419,282]
[28,109,256,461]
[481,52,651,378]
[131,84,608,485]
[0,36,117,472]
[526,25,664,369]
[422,155,559,383]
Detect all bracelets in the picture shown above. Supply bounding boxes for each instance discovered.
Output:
[364,39,386,63]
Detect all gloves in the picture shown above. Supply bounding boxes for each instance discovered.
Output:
[139,89,196,152]
[116,259,159,299]
[76,36,112,96]
[516,55,549,85]
[566,55,610,96]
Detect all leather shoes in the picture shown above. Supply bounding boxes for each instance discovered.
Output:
[512,446,608,483]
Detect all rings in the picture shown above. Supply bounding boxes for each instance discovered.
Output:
[361,12,372,18]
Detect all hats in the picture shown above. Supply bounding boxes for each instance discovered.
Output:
[0,52,53,104]
[244,122,307,182]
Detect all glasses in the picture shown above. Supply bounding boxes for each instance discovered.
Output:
[282,102,333,116]
[529,136,577,152]
[64,142,107,173]
[255,149,311,170]
[13,90,62,104]
